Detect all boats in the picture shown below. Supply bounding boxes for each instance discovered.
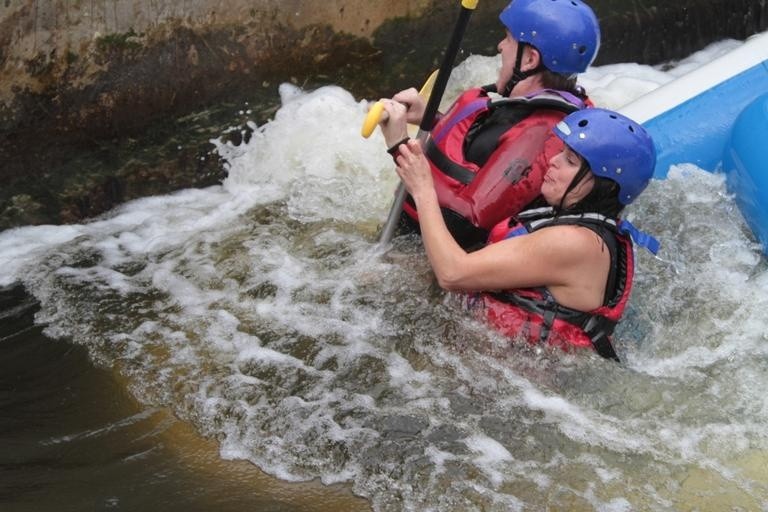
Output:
[614,31,768,272]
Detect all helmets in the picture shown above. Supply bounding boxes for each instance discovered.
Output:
[498,1,602,97]
[553,108,657,206]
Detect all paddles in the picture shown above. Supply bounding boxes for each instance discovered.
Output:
[360,68,438,140]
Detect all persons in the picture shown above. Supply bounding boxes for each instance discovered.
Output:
[396,106,656,365]
[362,0,600,251]
